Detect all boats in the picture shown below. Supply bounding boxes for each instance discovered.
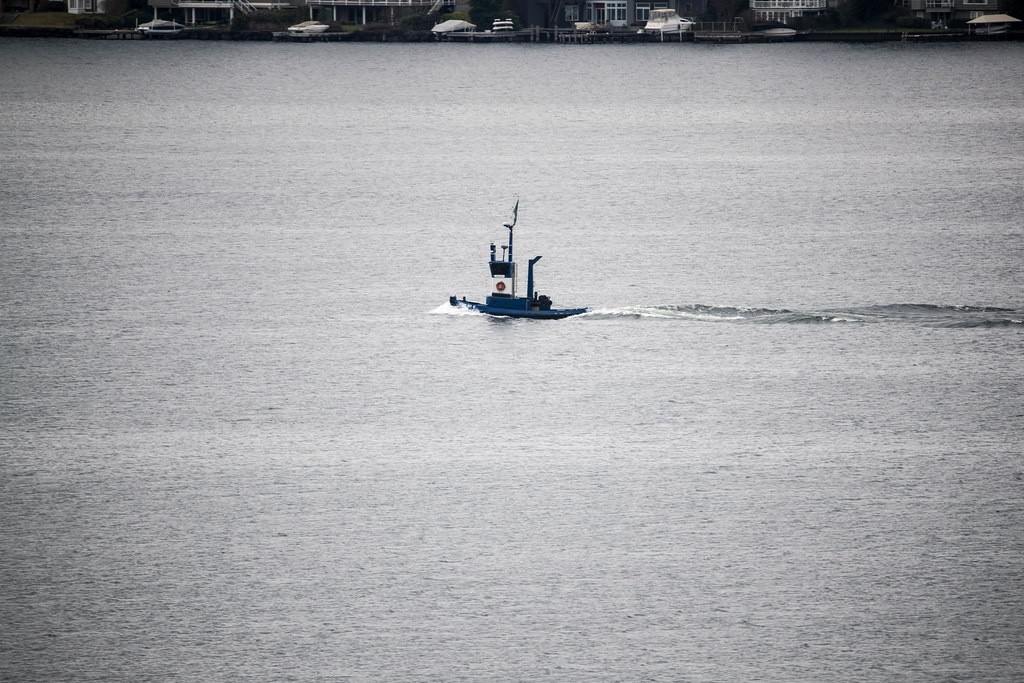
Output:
[450,198,588,323]
